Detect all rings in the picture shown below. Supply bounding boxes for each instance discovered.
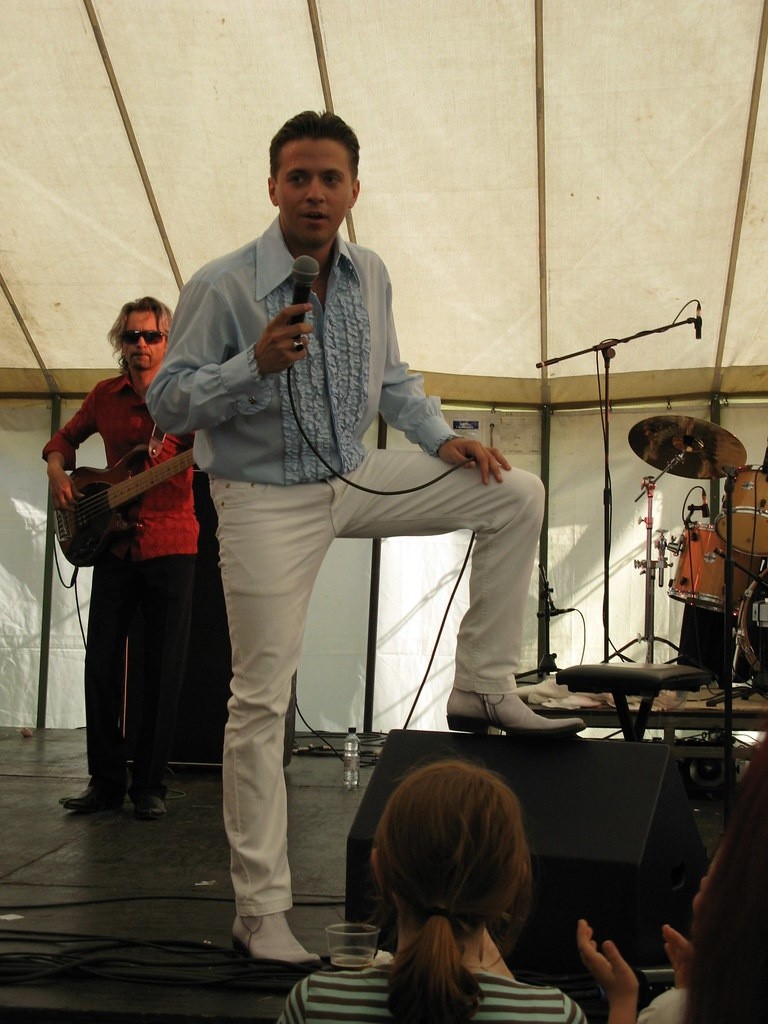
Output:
[293,338,304,352]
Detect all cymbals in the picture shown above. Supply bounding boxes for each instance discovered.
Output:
[629,414,748,481]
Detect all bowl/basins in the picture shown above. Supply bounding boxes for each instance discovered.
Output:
[325,924,379,968]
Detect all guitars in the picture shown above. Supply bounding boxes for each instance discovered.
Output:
[55,447,196,566]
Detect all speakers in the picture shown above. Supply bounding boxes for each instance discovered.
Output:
[123,473,296,771]
[345,728,709,983]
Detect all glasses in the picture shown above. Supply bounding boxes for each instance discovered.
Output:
[120,329,164,346]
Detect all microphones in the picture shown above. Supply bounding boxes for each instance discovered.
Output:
[702,489,709,518]
[286,255,319,340]
[696,304,703,339]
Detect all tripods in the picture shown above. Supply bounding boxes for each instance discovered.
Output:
[514,318,768,707]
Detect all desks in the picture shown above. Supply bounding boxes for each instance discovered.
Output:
[486,671,768,742]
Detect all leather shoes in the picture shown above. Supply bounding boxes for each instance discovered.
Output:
[135,792,168,820]
[446,688,587,738]
[63,782,124,813]
[232,909,323,967]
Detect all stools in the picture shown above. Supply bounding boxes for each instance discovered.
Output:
[556,664,709,741]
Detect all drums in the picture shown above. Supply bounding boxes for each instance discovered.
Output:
[669,520,760,616]
[737,569,768,672]
[713,464,768,555]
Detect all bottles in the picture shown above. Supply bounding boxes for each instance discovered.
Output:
[343,728,360,788]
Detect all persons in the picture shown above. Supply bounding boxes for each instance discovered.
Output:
[275,739,768,1024]
[43,297,200,817]
[143,110,586,969]
